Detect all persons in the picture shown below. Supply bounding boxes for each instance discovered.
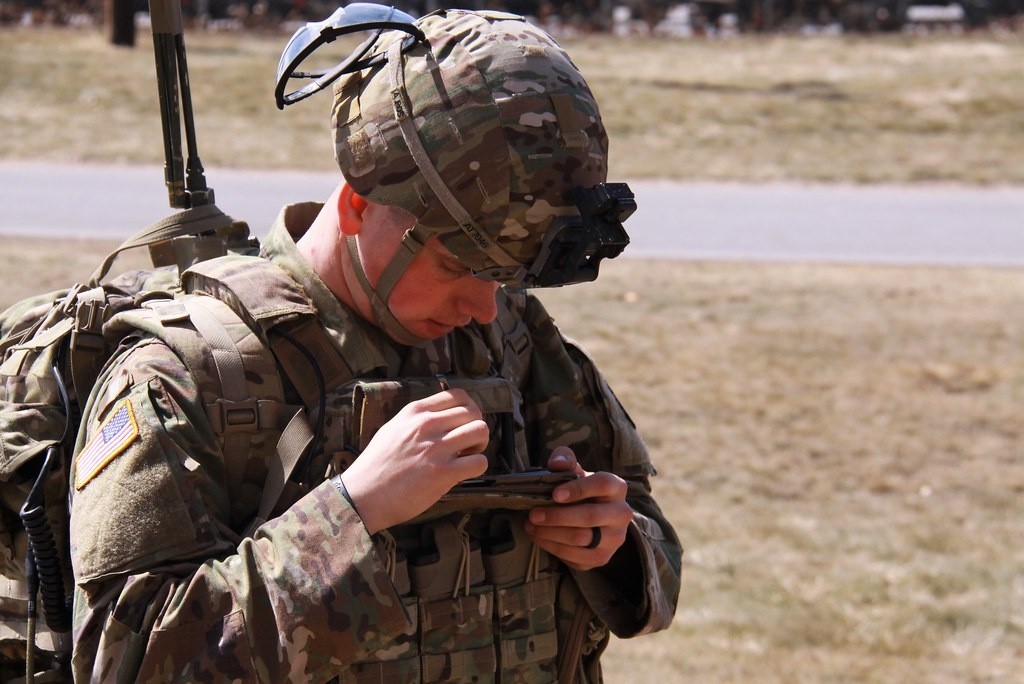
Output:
[70,4,685,684]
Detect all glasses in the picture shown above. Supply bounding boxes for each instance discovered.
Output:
[273,2,425,111]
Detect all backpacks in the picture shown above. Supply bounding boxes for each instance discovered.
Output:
[0,201,537,683]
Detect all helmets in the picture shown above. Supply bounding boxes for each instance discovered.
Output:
[331,6,612,291]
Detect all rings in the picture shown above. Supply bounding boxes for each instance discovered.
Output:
[584,527,602,549]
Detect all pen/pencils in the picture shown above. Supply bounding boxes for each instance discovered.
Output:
[434,374,449,390]
